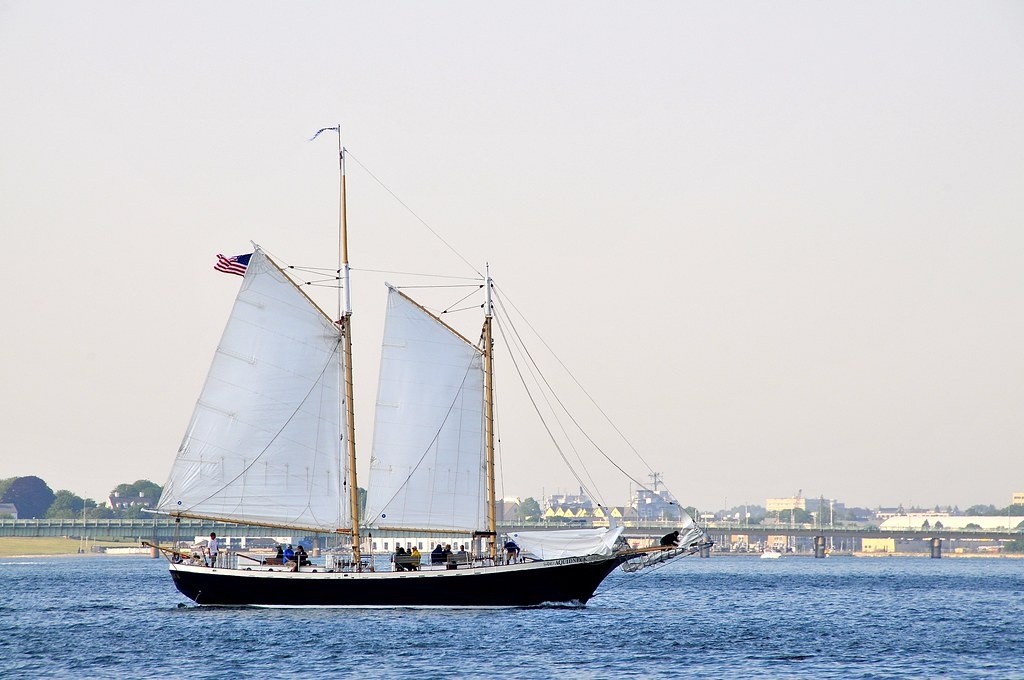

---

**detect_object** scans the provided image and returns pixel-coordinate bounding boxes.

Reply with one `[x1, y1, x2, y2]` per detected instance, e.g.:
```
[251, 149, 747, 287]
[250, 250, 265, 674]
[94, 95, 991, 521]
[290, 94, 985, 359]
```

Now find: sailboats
[141, 127, 711, 612]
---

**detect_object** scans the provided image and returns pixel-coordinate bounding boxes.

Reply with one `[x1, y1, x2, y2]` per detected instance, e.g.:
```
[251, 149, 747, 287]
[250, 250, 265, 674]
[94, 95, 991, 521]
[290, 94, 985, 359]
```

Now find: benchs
[264, 558, 298, 572]
[390, 552, 473, 572]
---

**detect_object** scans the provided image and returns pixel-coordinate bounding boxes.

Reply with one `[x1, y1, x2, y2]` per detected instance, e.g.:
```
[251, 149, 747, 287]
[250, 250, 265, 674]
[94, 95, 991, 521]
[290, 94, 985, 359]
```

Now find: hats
[276, 544, 282, 548]
[412, 546, 417, 549]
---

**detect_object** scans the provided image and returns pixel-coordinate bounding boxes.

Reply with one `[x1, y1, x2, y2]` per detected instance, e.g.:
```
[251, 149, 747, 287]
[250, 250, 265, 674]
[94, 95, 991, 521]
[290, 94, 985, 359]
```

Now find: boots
[513, 558, 518, 564]
[506, 560, 509, 565]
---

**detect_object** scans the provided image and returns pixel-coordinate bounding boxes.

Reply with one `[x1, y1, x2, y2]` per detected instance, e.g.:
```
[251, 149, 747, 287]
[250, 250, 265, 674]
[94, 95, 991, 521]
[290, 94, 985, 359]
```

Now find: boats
[761, 552, 781, 558]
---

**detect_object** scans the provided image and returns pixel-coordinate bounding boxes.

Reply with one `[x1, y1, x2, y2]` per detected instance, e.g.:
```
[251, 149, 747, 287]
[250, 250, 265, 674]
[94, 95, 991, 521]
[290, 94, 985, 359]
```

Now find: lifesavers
[173, 553, 181, 563]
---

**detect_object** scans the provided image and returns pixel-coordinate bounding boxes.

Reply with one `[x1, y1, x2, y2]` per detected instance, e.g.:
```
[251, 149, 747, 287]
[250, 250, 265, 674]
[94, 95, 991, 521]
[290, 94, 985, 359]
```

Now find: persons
[207, 532, 220, 568]
[488, 542, 496, 558]
[660, 530, 681, 551]
[390, 546, 421, 571]
[503, 541, 521, 565]
[275, 544, 313, 572]
[430, 543, 454, 563]
[458, 544, 468, 556]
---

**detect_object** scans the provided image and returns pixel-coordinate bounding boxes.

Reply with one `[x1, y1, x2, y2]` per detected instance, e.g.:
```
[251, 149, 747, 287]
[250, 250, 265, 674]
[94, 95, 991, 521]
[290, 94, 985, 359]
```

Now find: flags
[212, 253, 254, 277]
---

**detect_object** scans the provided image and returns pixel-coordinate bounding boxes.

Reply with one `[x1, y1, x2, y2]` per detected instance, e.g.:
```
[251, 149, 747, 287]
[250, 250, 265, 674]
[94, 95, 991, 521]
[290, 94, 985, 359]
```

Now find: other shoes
[668, 557, 673, 559]
[659, 560, 663, 562]
